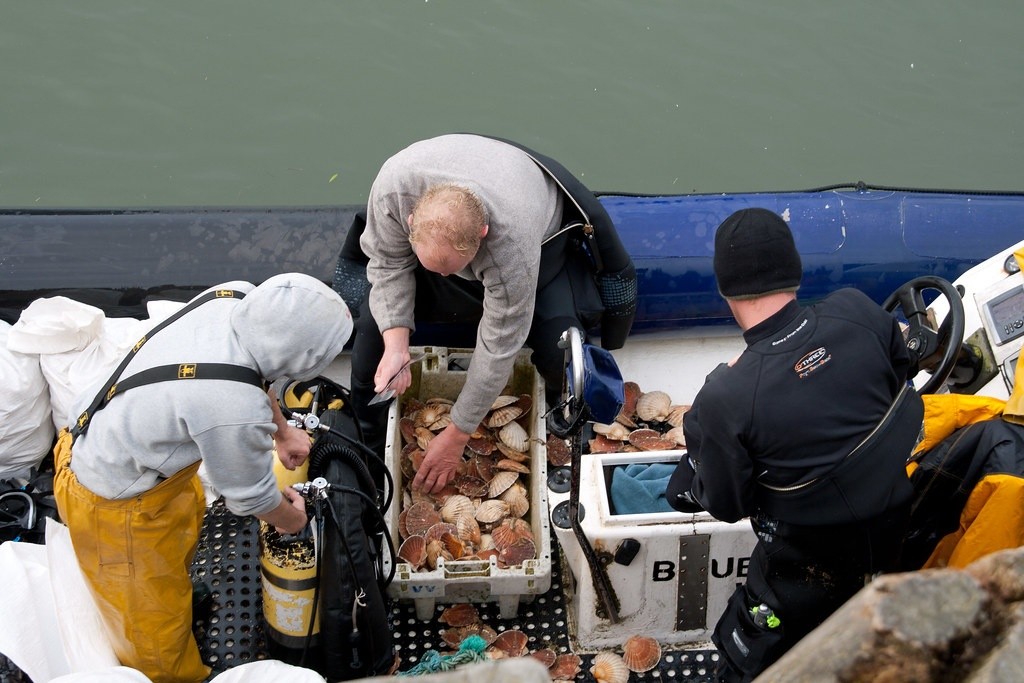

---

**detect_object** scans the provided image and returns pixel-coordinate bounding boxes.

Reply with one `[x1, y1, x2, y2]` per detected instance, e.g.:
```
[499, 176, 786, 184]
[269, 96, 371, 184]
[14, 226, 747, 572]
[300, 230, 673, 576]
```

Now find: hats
[713, 207, 803, 300]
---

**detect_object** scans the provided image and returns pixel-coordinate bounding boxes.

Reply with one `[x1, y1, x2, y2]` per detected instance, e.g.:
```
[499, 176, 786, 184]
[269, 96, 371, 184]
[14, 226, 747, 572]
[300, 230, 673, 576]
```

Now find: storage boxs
[546, 449, 758, 647]
[378, 344, 554, 618]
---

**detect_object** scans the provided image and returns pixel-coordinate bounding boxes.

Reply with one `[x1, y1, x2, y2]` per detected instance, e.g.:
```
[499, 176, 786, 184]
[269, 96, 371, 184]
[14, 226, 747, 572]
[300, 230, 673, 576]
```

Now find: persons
[50, 272, 353, 683]
[350, 132, 638, 493]
[667, 207, 924, 683]
